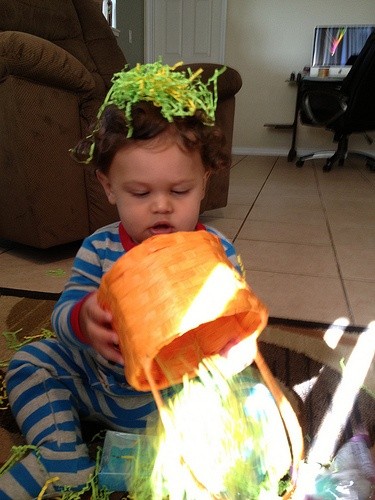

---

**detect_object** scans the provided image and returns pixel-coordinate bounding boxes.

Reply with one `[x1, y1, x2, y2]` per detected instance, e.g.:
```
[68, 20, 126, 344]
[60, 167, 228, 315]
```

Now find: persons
[0, 61, 305, 500]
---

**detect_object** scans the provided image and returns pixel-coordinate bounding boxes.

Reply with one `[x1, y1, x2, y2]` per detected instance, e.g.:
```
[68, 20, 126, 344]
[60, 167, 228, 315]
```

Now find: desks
[285, 76, 344, 164]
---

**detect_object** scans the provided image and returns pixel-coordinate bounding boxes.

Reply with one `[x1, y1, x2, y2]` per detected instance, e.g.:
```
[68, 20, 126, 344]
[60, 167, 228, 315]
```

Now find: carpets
[0, 285, 375, 499]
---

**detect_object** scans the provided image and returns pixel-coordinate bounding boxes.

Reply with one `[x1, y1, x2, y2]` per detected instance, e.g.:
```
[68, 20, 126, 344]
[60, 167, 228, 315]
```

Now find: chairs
[299, 29, 375, 173]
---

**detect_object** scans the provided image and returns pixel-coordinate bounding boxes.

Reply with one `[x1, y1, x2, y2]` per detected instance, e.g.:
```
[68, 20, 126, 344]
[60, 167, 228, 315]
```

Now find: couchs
[0, 0, 243, 249]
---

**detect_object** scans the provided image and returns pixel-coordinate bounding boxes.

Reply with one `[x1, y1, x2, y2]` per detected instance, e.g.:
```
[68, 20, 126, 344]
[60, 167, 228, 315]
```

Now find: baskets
[95, 231, 304, 499]
